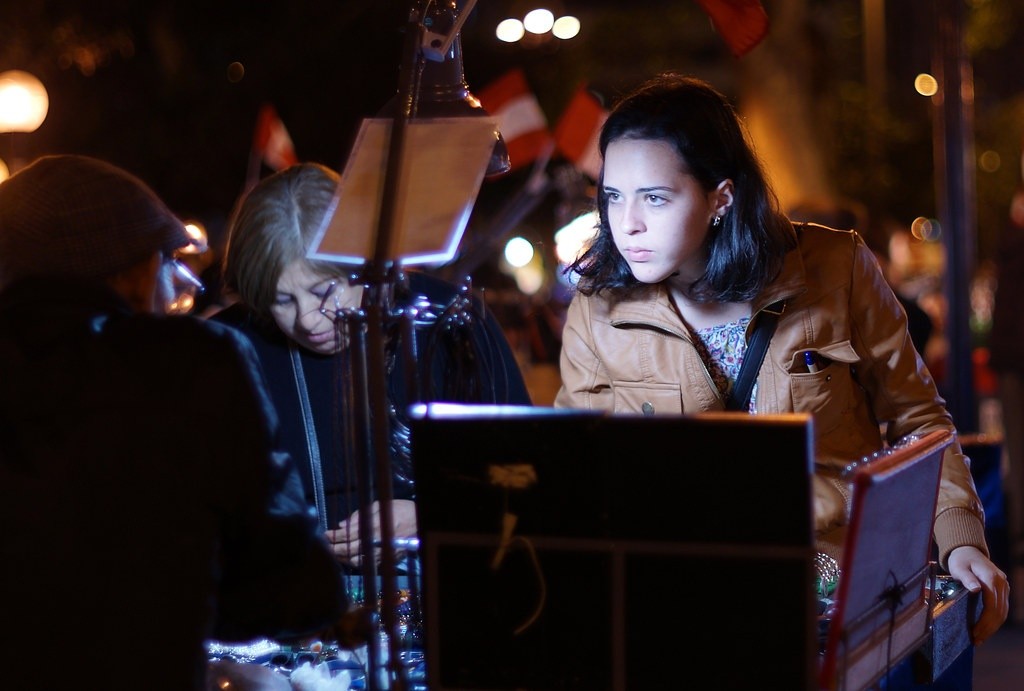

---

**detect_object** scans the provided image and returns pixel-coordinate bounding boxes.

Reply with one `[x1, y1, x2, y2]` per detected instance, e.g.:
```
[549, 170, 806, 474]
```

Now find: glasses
[165, 254, 206, 314]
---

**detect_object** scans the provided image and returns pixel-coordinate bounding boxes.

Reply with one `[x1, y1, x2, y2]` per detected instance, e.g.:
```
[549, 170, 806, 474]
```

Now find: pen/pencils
[805, 352, 818, 373]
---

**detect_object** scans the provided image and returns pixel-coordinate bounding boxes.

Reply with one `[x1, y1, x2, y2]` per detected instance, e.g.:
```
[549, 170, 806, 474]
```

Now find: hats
[0, 155, 191, 275]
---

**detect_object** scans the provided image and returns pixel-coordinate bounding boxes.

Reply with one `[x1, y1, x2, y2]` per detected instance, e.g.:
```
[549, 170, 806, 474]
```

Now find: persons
[0, 155, 347, 691]
[552, 71, 1010, 647]
[208, 162, 534, 576]
